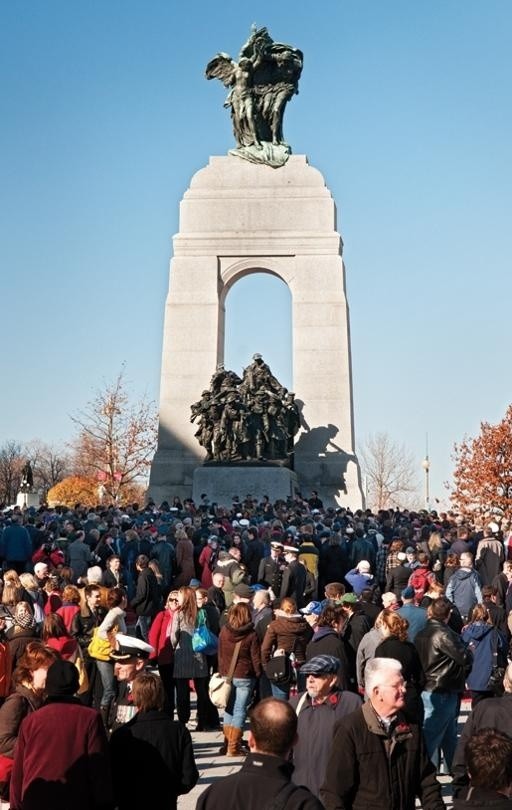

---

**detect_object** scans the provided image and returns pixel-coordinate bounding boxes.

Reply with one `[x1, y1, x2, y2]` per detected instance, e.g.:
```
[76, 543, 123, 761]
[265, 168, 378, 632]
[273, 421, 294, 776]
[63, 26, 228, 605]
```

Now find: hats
[238, 518, 250, 529]
[335, 593, 358, 606]
[270, 540, 284, 552]
[107, 632, 156, 662]
[401, 585, 417, 599]
[298, 654, 341, 676]
[282, 546, 300, 556]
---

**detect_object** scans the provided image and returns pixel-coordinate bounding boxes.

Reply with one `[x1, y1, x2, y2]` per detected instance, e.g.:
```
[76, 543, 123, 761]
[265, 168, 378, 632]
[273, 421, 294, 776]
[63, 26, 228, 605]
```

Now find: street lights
[422, 432, 432, 512]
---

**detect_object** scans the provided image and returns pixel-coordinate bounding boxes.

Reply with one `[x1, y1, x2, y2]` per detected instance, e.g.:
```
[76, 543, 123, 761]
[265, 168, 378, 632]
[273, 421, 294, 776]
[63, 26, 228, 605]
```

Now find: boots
[227, 727, 251, 757]
[219, 725, 233, 755]
[99, 704, 113, 735]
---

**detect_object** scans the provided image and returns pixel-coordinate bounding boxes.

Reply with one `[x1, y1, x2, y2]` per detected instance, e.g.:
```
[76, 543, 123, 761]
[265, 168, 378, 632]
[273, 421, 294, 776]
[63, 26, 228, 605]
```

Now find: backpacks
[411, 570, 433, 600]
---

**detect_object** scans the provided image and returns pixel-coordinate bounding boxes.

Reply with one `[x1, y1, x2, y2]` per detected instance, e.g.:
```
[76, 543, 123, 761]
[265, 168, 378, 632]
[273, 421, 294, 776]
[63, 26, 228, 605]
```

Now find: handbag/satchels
[485, 663, 507, 696]
[191, 624, 219, 655]
[208, 673, 233, 708]
[262, 656, 295, 686]
[88, 626, 118, 661]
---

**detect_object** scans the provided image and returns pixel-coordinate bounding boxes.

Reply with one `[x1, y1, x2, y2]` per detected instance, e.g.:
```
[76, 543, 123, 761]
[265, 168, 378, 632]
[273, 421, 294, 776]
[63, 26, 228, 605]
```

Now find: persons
[262, 43, 304, 144]
[191, 353, 308, 464]
[2, 487, 511, 809]
[218, 37, 266, 150]
[22, 459, 34, 488]
